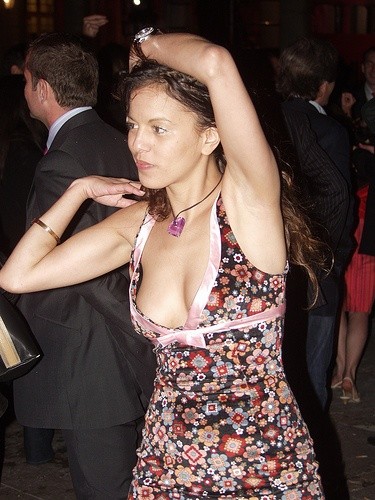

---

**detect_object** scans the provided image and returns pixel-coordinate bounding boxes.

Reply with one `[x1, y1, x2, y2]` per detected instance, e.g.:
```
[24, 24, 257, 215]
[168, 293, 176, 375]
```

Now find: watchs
[133, 25, 164, 65]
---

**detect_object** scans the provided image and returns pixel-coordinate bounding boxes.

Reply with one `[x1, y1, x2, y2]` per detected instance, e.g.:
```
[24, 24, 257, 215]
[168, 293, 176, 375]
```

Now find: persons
[1, 23, 325, 500]
[1, 13, 375, 466]
[12, 31, 156, 499]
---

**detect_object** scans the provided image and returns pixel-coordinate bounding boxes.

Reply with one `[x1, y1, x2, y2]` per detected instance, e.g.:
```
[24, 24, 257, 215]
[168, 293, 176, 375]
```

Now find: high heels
[340, 377, 361, 405]
[331, 376, 343, 392]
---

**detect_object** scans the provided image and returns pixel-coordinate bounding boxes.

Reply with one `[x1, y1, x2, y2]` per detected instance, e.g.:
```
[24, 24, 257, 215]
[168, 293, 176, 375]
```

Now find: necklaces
[162, 167, 226, 239]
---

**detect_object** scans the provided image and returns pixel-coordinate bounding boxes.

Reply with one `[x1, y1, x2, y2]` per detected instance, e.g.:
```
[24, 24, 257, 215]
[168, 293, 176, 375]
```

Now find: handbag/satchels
[0, 291, 41, 383]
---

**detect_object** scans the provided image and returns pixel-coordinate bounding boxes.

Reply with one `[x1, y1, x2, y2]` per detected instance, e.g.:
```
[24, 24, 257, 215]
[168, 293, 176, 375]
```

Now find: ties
[43, 145, 48, 157]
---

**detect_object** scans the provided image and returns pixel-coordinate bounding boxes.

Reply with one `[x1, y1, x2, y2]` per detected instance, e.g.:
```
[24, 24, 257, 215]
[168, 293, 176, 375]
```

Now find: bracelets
[31, 217, 60, 245]
